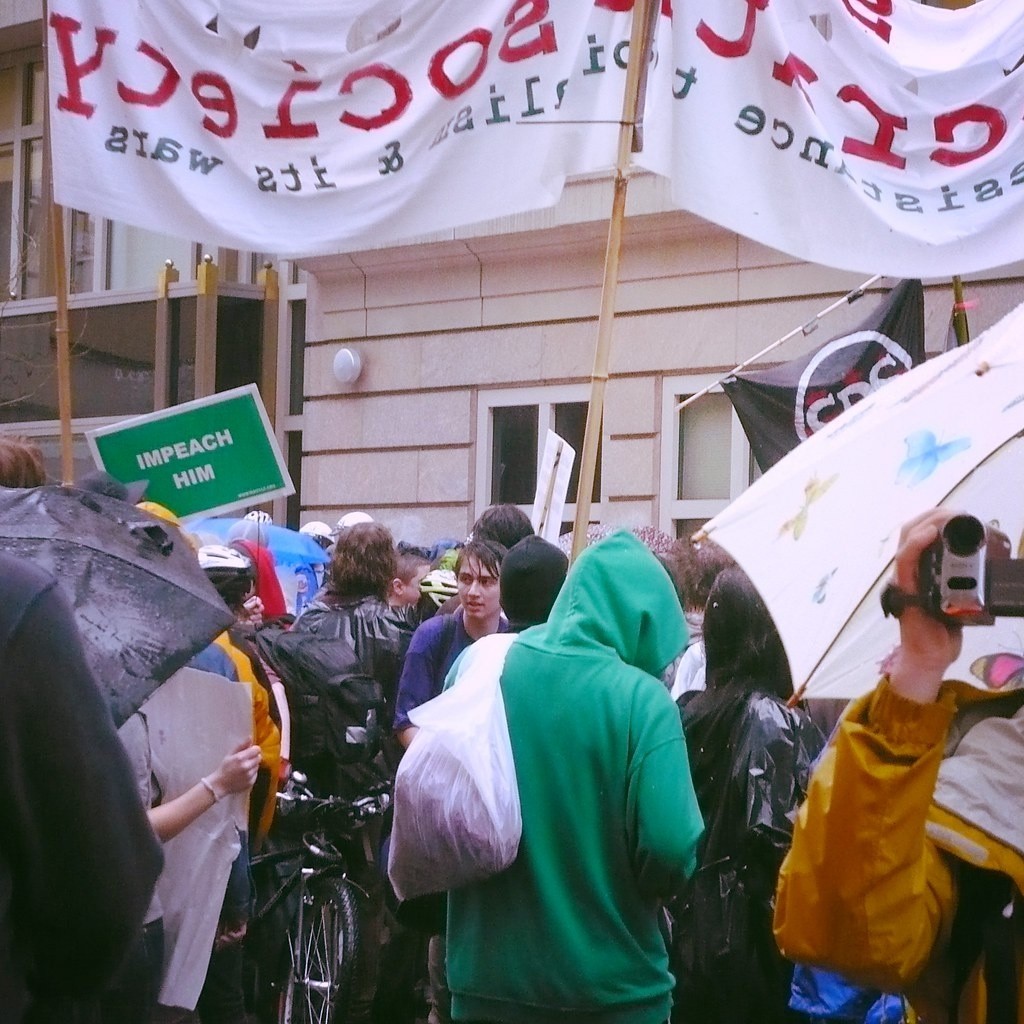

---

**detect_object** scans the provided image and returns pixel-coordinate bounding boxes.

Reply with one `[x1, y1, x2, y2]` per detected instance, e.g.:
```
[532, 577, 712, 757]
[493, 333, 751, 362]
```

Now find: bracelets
[201, 778, 220, 804]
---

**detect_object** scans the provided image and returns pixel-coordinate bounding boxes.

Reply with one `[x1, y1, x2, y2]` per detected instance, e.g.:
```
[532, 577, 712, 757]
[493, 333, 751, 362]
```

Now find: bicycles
[243, 767, 398, 1024]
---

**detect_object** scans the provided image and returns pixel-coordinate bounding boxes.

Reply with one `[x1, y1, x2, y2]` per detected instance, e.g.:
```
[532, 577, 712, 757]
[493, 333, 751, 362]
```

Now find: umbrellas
[186, 518, 331, 567]
[692, 300, 1023, 706]
[0, 485, 239, 731]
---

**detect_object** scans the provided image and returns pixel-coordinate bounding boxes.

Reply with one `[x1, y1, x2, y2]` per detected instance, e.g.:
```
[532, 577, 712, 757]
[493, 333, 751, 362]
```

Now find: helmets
[243, 509, 273, 525]
[435, 548, 460, 570]
[417, 569, 459, 608]
[134, 500, 181, 527]
[197, 544, 252, 569]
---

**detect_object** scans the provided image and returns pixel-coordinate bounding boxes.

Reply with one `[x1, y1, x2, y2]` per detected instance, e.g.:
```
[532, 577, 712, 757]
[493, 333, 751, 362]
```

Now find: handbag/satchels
[385, 633, 525, 903]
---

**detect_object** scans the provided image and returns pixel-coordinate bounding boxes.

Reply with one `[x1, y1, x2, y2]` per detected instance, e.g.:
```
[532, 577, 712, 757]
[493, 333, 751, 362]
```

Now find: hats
[329, 511, 375, 536]
[299, 521, 335, 544]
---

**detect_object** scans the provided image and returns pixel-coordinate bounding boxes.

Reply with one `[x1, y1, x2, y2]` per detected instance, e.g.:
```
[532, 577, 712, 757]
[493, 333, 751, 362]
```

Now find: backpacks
[228, 538, 290, 624]
[246, 622, 383, 768]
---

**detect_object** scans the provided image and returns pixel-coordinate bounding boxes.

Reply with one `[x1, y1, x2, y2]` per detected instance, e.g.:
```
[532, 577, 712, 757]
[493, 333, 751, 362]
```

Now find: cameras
[917, 514, 1024, 627]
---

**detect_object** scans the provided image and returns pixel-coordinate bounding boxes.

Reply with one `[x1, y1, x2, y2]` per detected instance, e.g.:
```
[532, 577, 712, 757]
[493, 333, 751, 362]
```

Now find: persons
[0, 436, 1024, 1024]
[443, 532, 705, 1024]
